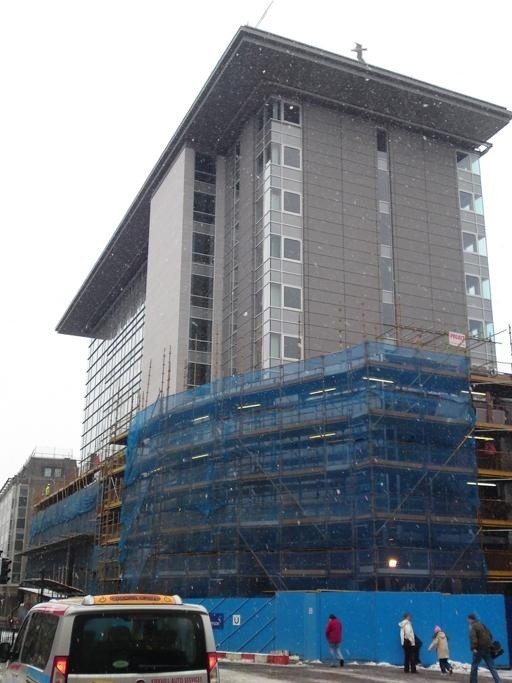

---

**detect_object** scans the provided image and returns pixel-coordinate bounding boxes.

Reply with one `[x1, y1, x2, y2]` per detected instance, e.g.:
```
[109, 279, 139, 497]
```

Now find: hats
[434, 624, 440, 632]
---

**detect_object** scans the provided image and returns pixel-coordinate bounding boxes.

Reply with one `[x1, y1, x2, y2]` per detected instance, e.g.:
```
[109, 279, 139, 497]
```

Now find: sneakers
[448, 668, 452, 676]
[411, 670, 419, 673]
[329, 665, 337, 667]
[340, 660, 344, 666]
[440, 672, 448, 676]
[403, 670, 410, 673]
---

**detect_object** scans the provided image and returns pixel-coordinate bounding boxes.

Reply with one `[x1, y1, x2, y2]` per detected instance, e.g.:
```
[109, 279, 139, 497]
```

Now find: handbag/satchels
[491, 641, 504, 658]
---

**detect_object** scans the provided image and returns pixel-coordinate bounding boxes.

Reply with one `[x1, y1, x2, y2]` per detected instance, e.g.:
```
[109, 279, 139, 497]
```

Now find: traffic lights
[0, 557, 11, 585]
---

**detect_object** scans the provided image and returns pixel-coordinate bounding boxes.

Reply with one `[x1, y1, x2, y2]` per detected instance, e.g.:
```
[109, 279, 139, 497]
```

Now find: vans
[0, 591, 219, 682]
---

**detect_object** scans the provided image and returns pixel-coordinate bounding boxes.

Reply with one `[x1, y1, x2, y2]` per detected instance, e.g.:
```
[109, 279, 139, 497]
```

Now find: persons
[467, 614, 502, 682]
[324, 614, 344, 667]
[46, 483, 52, 496]
[426, 625, 453, 677]
[397, 612, 420, 673]
